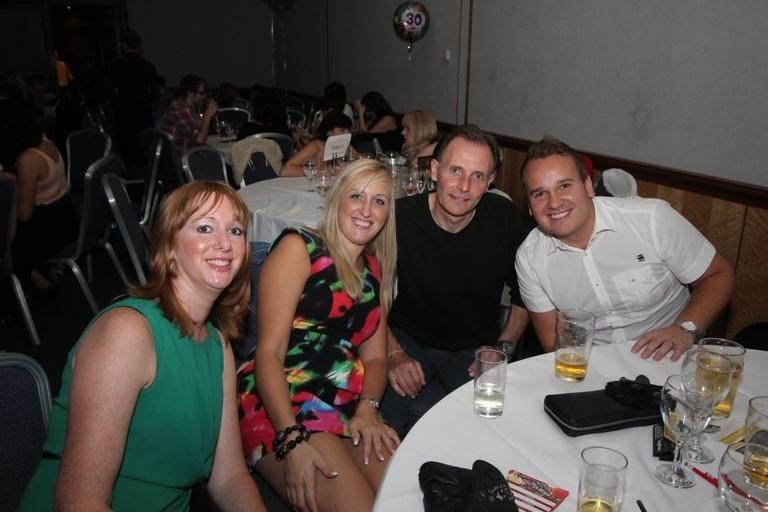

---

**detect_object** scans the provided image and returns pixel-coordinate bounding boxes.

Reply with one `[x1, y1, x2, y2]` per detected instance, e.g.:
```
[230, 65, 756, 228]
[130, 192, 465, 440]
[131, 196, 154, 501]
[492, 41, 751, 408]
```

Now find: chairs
[731, 322, 768, 349]
[592, 169, 636, 200]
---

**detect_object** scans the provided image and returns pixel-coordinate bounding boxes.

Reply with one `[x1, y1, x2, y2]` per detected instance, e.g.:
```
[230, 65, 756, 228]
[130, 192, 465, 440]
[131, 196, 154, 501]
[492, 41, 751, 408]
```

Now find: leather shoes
[28, 278, 57, 298]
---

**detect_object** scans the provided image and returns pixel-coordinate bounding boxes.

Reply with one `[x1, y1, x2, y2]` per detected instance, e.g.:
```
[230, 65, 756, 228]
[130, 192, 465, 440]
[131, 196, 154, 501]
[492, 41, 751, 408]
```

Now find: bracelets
[359, 396, 380, 408]
[387, 350, 405, 359]
[271, 424, 310, 462]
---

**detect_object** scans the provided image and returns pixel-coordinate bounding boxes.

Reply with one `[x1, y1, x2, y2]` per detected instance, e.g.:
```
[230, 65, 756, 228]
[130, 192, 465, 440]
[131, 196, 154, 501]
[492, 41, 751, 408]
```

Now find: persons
[164, 72, 439, 198]
[235, 158, 401, 512]
[383, 123, 530, 436]
[514, 134, 737, 361]
[13, 181, 267, 512]
[2, 28, 161, 305]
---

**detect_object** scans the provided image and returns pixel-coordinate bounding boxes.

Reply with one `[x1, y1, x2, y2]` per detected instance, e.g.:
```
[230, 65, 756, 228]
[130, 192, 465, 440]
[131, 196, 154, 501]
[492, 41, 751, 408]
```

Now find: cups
[472, 348, 509, 419]
[576, 446, 629, 512]
[553, 309, 597, 383]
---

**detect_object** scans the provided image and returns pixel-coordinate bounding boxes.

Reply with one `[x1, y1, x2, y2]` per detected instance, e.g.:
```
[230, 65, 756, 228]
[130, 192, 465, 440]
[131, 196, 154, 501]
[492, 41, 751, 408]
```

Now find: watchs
[493, 340, 517, 359]
[673, 319, 705, 340]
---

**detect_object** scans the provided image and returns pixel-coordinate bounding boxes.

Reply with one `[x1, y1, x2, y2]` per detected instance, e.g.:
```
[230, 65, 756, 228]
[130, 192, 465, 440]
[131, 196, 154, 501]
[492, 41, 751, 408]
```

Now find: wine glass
[652, 335, 768, 512]
[301, 148, 436, 211]
[205, 97, 251, 145]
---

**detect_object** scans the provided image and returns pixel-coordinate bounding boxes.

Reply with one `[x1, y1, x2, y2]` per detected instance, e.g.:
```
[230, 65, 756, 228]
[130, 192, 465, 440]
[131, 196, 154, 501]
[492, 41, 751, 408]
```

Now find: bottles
[372, 138, 389, 166]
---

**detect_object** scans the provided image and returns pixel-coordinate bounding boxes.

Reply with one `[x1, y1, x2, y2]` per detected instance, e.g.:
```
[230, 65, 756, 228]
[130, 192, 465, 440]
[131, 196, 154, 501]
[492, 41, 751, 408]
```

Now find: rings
[666, 340, 674, 348]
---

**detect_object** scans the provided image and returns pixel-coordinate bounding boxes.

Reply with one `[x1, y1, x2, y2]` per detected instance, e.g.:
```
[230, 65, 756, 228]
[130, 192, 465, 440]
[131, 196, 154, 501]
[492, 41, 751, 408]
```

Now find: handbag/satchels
[418, 461, 520, 512]
[541, 385, 664, 438]
